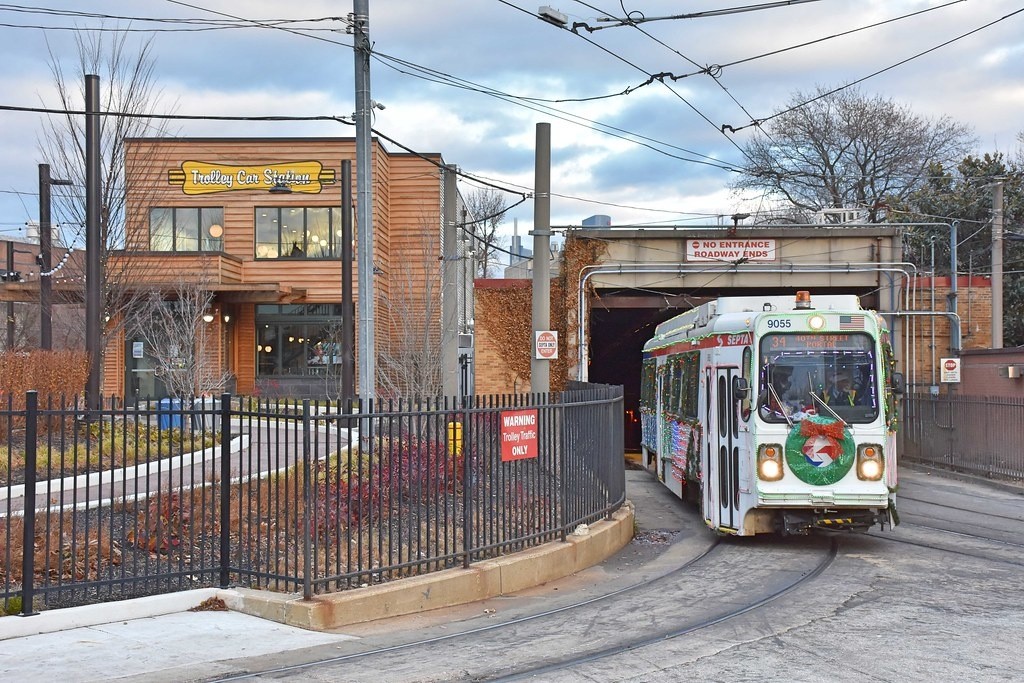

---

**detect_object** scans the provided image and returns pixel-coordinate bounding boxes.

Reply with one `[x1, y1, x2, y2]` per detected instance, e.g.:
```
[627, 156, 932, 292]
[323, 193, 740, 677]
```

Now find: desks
[308, 367, 331, 375]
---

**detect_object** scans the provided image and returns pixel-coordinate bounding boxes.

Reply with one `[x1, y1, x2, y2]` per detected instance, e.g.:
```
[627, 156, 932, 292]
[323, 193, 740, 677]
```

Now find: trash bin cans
[155, 398, 190, 433]
[192, 397, 221, 432]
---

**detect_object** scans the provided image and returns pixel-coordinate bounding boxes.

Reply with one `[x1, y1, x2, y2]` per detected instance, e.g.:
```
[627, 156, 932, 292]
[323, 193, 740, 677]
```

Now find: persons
[815, 374, 861, 406]
[315, 338, 328, 364]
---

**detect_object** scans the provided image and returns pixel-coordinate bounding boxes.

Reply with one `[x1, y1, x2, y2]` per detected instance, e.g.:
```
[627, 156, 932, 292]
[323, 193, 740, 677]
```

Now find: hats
[829, 372, 848, 383]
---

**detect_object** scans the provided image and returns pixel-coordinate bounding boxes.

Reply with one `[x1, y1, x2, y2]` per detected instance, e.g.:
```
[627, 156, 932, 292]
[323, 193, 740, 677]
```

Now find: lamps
[202, 310, 213, 322]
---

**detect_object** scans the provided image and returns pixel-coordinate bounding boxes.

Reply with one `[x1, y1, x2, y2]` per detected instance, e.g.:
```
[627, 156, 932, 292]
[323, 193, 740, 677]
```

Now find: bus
[639, 290, 904, 536]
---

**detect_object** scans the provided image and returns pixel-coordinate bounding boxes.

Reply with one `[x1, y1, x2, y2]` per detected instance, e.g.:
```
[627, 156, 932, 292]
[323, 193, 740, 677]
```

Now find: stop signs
[944, 360, 958, 371]
[537, 330, 558, 358]
[692, 240, 699, 249]
